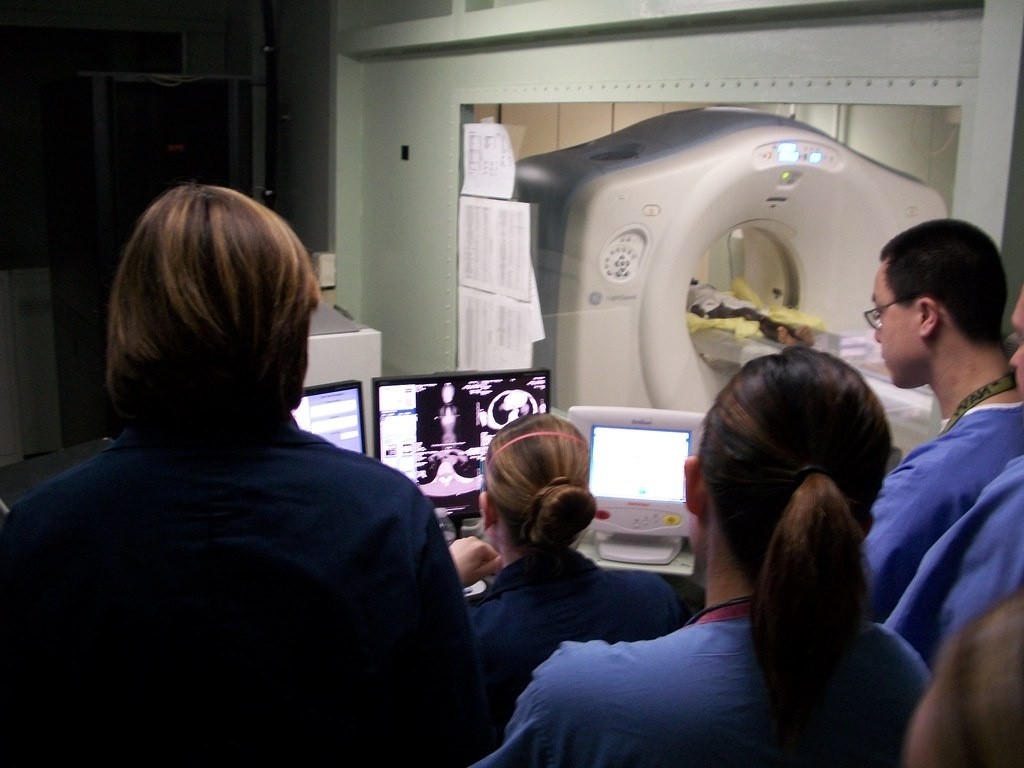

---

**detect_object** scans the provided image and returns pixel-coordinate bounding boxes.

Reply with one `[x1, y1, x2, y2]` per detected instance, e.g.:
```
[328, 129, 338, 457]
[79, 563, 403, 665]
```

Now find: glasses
[864, 295, 944, 330]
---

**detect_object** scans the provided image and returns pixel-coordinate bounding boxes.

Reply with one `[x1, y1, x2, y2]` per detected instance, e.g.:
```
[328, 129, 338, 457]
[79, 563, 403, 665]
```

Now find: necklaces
[696, 602, 752, 624]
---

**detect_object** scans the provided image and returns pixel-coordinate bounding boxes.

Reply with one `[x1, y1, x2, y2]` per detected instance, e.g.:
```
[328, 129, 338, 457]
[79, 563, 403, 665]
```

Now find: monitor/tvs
[291, 379, 367, 456]
[372, 368, 551, 541]
[567, 406, 707, 565]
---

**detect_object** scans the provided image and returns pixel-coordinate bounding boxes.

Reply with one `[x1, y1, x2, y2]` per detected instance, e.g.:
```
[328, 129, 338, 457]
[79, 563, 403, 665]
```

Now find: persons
[686, 278, 817, 347]
[865, 218, 1024, 768]
[0, 185, 497, 768]
[450, 347, 934, 768]
